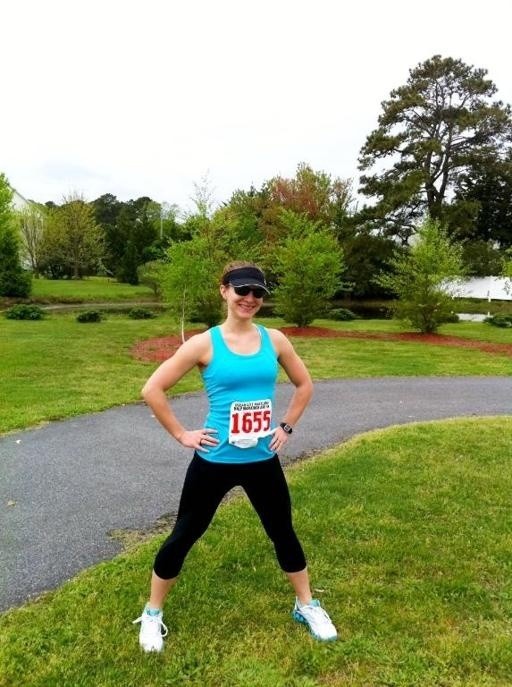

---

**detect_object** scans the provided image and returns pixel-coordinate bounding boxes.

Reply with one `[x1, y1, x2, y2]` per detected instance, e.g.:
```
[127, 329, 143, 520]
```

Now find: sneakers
[138, 601, 164, 655]
[291, 595, 339, 643]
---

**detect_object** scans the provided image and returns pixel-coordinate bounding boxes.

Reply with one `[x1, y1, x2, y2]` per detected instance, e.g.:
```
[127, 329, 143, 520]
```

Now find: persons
[131, 258, 340, 656]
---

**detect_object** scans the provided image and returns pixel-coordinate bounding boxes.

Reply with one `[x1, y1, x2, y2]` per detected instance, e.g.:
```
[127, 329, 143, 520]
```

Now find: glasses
[227, 284, 268, 299]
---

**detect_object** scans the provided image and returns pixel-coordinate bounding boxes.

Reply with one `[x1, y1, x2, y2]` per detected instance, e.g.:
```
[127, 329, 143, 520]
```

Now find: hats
[221, 267, 271, 296]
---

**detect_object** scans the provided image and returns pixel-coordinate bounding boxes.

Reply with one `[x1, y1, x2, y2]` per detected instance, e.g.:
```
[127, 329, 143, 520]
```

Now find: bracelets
[279, 422, 293, 434]
